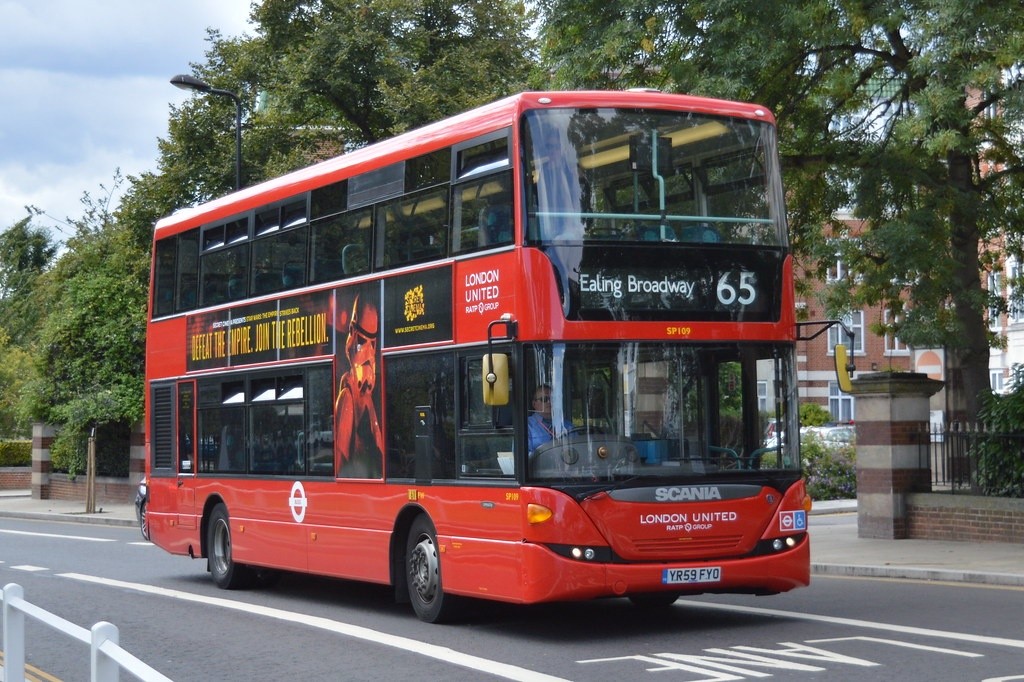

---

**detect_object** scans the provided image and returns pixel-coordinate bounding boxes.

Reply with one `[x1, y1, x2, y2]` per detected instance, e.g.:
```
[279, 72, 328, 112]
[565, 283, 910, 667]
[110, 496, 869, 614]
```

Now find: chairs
[169, 189, 719, 307]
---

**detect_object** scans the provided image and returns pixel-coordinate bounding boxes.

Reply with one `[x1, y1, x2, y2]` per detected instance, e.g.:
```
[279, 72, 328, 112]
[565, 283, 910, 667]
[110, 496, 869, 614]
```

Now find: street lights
[169, 72, 243, 193]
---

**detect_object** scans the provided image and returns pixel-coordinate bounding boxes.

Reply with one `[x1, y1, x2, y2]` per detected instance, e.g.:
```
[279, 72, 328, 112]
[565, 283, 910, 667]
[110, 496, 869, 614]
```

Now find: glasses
[536, 395, 550, 403]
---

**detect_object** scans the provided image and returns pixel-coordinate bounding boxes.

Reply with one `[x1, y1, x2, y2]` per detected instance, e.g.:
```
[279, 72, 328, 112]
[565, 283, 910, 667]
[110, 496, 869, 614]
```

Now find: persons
[528, 384, 578, 451]
[432, 371, 492, 479]
[335, 293, 381, 476]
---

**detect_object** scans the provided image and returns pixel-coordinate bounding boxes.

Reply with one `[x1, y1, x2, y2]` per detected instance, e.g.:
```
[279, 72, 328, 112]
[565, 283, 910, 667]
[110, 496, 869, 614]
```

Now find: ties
[548, 422, 554, 439]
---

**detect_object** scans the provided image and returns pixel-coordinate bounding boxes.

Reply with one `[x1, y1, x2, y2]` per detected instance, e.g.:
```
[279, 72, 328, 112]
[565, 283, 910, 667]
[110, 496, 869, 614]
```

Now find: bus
[145, 86, 857, 616]
